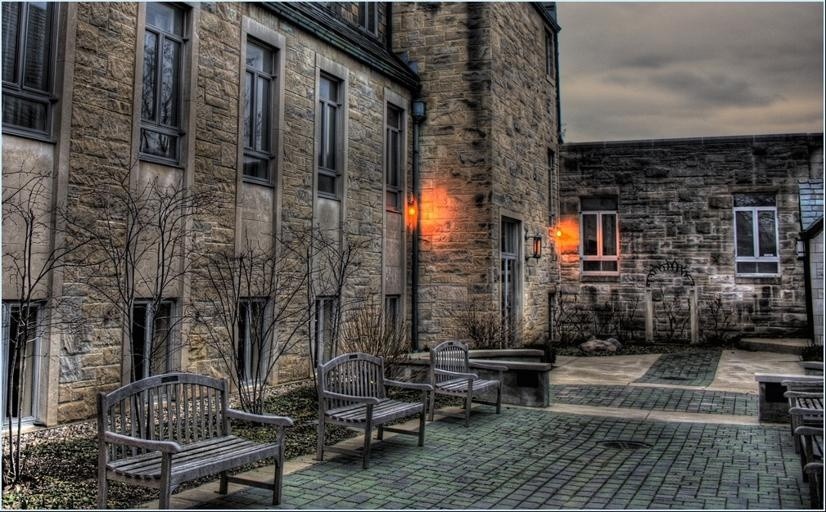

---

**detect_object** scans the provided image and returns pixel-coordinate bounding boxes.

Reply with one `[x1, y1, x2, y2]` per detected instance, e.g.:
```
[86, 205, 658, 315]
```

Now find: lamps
[524, 232, 542, 263]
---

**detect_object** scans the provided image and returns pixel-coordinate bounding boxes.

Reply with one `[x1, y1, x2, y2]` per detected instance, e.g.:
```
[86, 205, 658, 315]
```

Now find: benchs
[427, 341, 504, 430]
[95, 372, 295, 508]
[313, 349, 436, 470]
